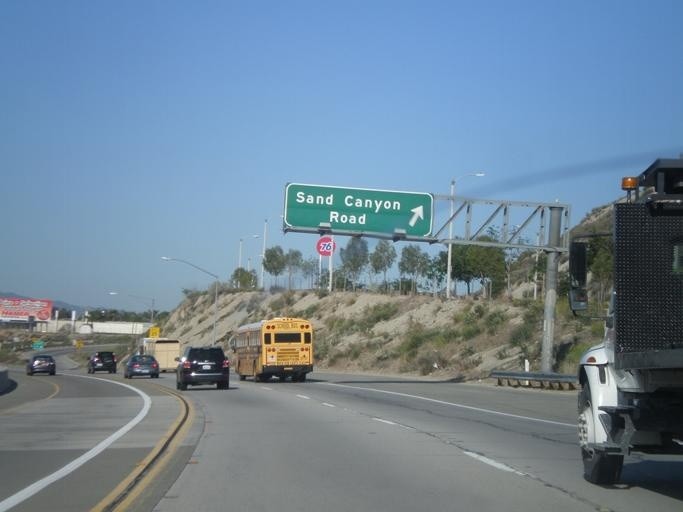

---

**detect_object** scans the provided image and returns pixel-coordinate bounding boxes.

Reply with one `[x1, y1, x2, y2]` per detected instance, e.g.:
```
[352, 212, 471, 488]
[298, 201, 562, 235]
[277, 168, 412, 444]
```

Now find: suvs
[86, 351, 116, 374]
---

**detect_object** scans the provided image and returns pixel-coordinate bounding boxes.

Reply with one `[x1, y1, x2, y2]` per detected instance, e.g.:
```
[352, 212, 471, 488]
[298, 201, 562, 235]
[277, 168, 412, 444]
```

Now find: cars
[25, 354, 55, 375]
[124, 355, 159, 379]
[173, 345, 229, 390]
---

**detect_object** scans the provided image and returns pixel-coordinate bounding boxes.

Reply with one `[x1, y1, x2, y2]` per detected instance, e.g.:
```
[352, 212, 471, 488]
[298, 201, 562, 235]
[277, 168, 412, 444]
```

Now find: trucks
[567, 179, 682, 485]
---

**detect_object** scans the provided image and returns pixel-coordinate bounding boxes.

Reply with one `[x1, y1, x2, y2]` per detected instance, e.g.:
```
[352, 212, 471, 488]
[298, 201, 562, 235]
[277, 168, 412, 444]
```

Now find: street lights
[445, 172, 485, 298]
[236, 215, 275, 289]
[107, 291, 155, 323]
[160, 256, 218, 346]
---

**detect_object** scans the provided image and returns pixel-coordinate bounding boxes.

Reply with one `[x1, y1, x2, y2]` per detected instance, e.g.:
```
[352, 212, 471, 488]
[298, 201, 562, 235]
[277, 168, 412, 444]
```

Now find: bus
[227, 317, 313, 382]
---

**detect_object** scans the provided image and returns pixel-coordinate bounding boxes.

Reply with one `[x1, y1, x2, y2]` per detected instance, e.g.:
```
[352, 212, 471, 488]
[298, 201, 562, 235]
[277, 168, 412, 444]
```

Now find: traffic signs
[281, 183, 433, 241]
